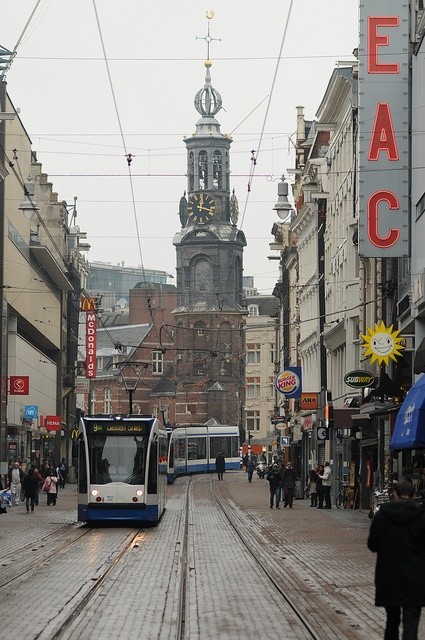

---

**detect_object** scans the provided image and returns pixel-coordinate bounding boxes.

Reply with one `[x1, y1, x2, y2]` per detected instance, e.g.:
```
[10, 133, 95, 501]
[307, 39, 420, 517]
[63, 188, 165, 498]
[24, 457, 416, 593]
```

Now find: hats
[394, 480, 416, 499]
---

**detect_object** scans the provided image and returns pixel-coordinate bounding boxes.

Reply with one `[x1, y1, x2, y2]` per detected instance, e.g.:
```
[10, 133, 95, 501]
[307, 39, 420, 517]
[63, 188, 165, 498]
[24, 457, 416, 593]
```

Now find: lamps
[17, 173, 40, 221]
[270, 173, 297, 222]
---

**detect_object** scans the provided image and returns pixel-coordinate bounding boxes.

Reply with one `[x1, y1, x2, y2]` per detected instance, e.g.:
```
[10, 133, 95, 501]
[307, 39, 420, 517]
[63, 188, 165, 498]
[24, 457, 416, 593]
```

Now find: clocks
[230, 193, 239, 225]
[185, 192, 216, 226]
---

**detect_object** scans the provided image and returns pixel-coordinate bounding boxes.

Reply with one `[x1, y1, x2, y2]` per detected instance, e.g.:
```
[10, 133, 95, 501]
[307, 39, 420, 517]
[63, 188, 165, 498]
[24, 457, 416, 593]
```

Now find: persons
[317, 465, 324, 509]
[367, 480, 425, 640]
[246, 458, 254, 482]
[307, 470, 318, 507]
[23, 457, 69, 513]
[280, 462, 297, 509]
[216, 452, 225, 480]
[266, 464, 282, 509]
[8, 461, 23, 506]
[318, 462, 332, 509]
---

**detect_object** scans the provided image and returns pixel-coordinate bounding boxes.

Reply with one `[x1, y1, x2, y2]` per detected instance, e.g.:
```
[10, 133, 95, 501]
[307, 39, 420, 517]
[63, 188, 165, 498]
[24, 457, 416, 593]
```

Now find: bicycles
[335, 475, 349, 510]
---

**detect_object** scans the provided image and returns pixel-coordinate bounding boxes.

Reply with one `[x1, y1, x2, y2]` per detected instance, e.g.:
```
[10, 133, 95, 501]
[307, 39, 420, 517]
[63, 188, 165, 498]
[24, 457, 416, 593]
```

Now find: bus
[74, 413, 243, 528]
